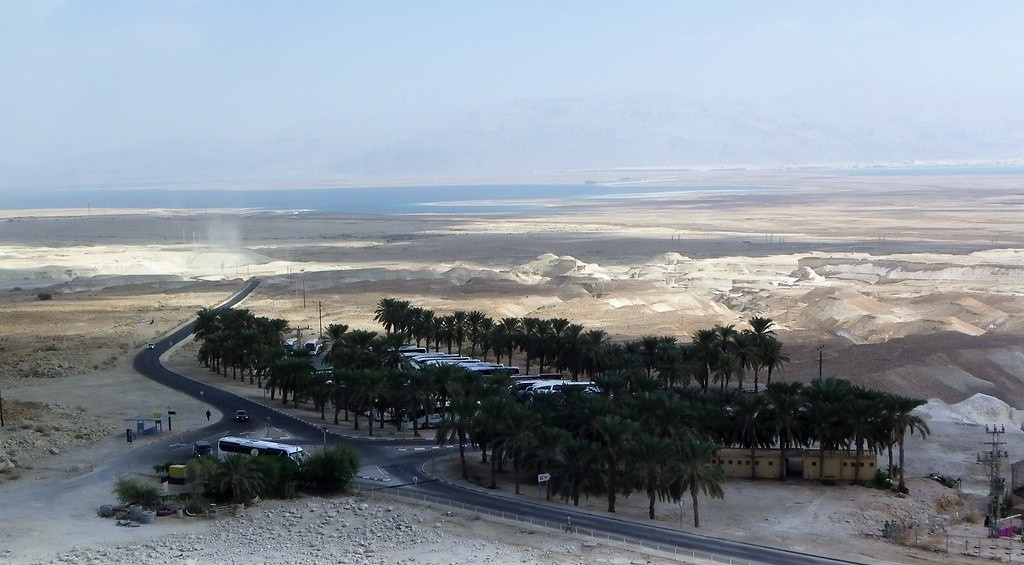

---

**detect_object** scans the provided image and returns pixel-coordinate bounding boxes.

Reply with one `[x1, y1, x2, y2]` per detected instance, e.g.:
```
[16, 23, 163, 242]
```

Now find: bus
[302, 339, 320, 356]
[368, 346, 598, 395]
[217, 436, 310, 471]
[283, 337, 297, 355]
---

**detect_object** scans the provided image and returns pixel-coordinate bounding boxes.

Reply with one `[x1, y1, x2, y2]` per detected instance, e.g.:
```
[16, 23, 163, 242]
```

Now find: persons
[206, 409, 211, 421]
[565, 512, 572, 534]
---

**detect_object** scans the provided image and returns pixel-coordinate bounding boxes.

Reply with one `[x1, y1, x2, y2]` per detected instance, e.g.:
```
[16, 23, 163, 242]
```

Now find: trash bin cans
[176, 508, 183, 519]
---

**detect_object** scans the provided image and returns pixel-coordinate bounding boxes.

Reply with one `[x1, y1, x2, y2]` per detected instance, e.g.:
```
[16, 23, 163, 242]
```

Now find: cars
[147, 343, 154, 348]
[234, 409, 249, 422]
[193, 440, 213, 459]
